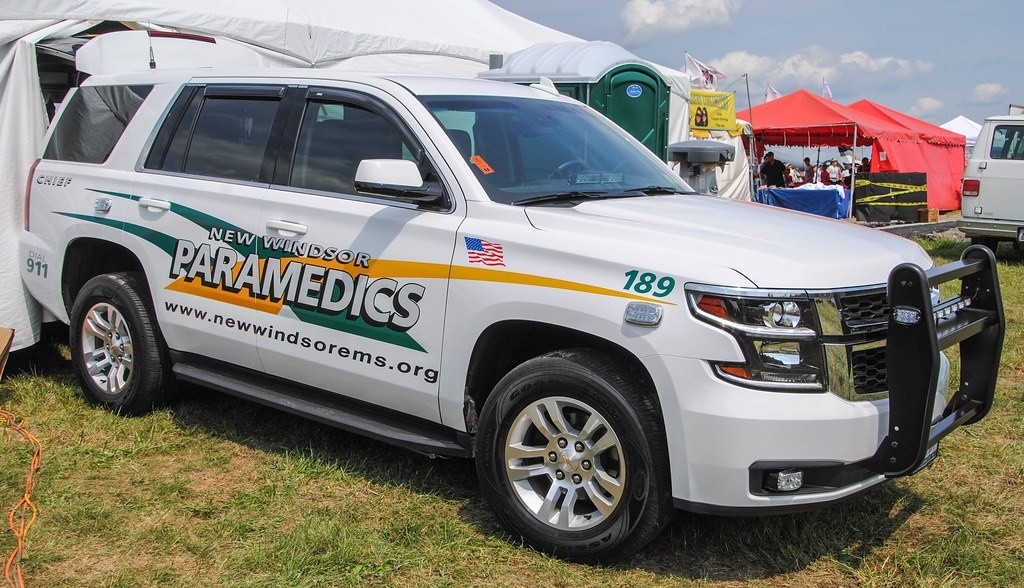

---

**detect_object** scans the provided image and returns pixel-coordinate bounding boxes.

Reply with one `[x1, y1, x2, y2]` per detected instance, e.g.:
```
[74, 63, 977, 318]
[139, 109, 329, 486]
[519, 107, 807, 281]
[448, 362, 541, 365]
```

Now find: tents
[1, 0, 757, 353]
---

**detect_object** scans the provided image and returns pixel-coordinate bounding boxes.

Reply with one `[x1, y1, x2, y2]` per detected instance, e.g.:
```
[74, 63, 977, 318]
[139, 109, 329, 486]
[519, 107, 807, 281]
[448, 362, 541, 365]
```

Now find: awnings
[938, 116, 1007, 149]
[736, 92, 965, 215]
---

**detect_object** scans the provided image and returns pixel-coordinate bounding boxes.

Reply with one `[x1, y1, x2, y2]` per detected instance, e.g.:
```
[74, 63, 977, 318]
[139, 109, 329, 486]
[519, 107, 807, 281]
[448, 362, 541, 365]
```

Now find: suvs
[21, 58, 1008, 565]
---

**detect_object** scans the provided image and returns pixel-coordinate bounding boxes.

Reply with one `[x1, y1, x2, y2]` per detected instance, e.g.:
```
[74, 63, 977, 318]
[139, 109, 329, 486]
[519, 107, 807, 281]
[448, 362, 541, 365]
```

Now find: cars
[955, 109, 1024, 263]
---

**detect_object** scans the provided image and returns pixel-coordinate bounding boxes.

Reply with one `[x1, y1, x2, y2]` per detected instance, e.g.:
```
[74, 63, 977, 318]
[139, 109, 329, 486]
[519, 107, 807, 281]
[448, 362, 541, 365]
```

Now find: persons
[757, 152, 791, 187]
[803, 157, 814, 182]
[813, 163, 824, 183]
[820, 165, 830, 183]
[760, 154, 771, 184]
[858, 157, 869, 171]
[828, 159, 842, 182]
[782, 160, 803, 186]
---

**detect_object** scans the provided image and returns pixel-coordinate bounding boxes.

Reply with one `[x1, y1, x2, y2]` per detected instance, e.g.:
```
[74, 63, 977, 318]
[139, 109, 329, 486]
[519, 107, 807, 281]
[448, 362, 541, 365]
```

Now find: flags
[767, 85, 781, 102]
[685, 53, 728, 92]
[823, 81, 832, 101]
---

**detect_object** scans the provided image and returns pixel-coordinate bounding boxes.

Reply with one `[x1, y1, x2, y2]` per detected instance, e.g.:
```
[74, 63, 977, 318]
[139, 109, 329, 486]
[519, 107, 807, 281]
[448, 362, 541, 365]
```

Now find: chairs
[186, 114, 247, 178]
[304, 119, 375, 200]
[416, 129, 472, 195]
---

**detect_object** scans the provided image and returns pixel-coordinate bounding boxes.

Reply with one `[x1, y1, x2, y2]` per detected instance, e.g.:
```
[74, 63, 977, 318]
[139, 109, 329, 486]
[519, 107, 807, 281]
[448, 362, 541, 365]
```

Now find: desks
[757, 186, 850, 222]
